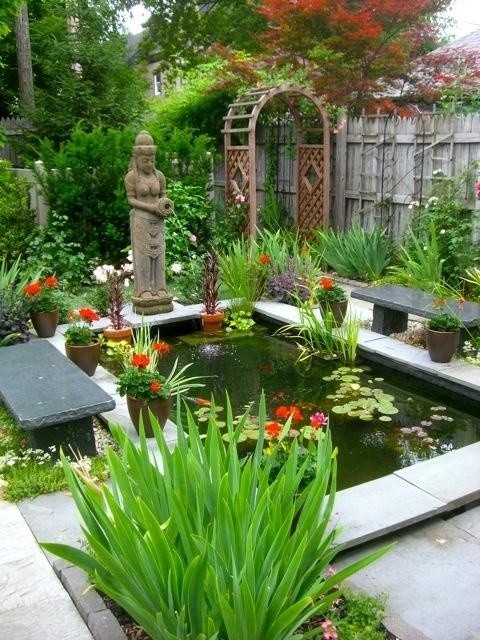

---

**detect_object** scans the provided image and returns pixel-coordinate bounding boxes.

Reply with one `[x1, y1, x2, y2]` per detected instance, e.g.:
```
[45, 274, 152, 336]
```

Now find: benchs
[0, 338, 117, 461]
[352, 283, 479, 355]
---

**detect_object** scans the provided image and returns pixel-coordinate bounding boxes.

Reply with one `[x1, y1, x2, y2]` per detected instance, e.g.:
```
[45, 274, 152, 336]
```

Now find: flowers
[62, 308, 99, 343]
[118, 346, 179, 398]
[316, 277, 347, 298]
[20, 274, 62, 310]
[258, 253, 269, 266]
[255, 406, 339, 489]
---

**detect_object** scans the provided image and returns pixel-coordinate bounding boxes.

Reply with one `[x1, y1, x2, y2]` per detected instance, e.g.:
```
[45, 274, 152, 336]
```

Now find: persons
[124, 129, 177, 306]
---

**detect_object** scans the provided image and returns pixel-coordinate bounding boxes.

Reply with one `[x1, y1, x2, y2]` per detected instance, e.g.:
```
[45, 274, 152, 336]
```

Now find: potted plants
[424, 314, 460, 363]
[102, 270, 133, 348]
[200, 252, 224, 332]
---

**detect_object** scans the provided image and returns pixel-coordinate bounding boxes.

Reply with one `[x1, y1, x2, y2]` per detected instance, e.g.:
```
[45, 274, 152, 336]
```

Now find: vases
[318, 298, 350, 327]
[276, 493, 308, 535]
[126, 399, 174, 439]
[65, 343, 100, 376]
[29, 311, 59, 336]
[242, 272, 266, 301]
